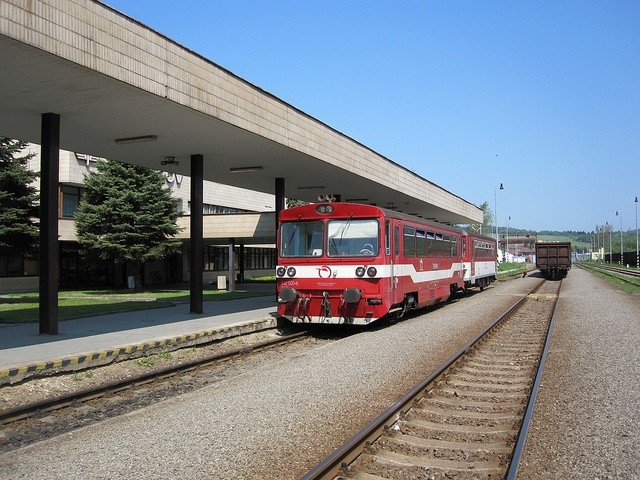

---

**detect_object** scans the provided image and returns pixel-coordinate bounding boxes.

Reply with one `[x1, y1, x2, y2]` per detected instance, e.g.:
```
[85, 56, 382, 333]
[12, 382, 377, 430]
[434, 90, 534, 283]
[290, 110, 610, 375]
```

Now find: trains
[535, 241, 571, 278]
[276, 195, 495, 332]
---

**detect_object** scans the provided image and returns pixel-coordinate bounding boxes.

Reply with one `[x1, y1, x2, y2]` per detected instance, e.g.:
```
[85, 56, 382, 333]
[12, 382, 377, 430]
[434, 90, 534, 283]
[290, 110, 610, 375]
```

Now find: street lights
[616, 211, 623, 266]
[496, 183, 504, 263]
[606, 221, 612, 264]
[506, 216, 511, 262]
[634, 196, 640, 268]
